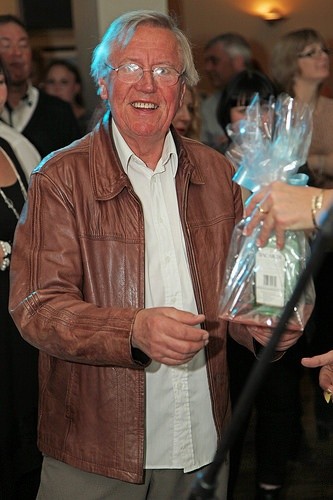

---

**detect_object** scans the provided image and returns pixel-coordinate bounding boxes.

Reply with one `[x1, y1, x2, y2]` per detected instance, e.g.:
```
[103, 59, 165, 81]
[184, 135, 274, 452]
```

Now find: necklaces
[0, 146, 28, 220]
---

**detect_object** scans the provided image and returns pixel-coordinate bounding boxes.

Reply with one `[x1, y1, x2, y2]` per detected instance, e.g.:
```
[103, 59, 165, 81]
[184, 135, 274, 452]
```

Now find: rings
[257, 204, 269, 214]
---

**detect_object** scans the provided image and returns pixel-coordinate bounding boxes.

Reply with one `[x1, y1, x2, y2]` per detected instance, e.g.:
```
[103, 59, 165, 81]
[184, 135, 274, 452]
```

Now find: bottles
[252, 233, 301, 318]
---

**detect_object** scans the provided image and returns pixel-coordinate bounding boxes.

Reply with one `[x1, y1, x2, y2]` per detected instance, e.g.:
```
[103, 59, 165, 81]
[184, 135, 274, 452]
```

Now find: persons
[266, 27, 333, 440]
[0, 15, 81, 160]
[45, 59, 94, 135]
[199, 31, 258, 155]
[171, 84, 202, 143]
[301, 350, 333, 404]
[8, 10, 303, 500]
[215, 69, 319, 500]
[0, 58, 42, 500]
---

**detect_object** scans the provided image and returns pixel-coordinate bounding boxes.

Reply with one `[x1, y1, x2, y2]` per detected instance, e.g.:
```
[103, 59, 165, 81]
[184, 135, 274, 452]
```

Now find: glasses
[105, 62, 184, 87]
[298, 46, 330, 57]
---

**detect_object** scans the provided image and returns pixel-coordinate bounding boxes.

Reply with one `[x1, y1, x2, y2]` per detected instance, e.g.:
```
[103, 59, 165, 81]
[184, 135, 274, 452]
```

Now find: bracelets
[0, 241, 7, 271]
[320, 155, 325, 175]
[311, 189, 324, 230]
